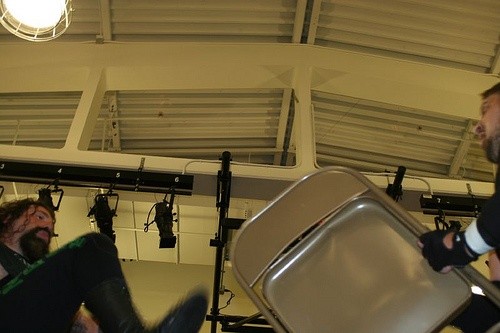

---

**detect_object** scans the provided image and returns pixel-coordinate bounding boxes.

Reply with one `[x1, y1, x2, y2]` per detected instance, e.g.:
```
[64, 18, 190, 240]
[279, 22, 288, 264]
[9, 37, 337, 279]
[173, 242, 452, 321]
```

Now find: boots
[84, 278, 207, 333]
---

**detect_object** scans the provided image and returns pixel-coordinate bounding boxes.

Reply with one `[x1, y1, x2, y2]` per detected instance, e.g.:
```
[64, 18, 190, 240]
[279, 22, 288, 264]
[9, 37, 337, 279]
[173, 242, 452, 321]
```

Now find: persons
[0, 198, 209, 333]
[419, 80, 500, 333]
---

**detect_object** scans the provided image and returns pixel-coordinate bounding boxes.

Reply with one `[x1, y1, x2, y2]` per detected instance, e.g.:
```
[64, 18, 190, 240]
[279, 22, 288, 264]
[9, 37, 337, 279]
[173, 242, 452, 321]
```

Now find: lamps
[0, 1, 73, 42]
[144, 190, 178, 249]
[36, 184, 63, 236]
[90, 183, 121, 244]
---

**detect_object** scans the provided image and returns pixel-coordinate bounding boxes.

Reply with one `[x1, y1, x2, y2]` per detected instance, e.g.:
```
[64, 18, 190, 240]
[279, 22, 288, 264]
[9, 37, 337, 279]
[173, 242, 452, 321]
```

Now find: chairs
[231, 166, 500, 333]
